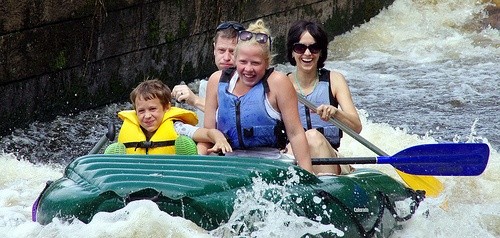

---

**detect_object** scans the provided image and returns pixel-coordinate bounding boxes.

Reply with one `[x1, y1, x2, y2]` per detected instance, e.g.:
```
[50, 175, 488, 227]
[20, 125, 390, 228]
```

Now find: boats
[30, 150, 427, 238]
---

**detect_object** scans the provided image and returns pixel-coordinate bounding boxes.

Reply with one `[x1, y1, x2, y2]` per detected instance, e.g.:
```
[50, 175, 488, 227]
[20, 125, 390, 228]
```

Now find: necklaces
[294, 69, 318, 97]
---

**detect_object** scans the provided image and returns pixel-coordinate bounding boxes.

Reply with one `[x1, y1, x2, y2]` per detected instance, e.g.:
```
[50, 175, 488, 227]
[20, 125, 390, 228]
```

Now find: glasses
[214, 23, 245, 33]
[292, 43, 321, 54]
[237, 30, 272, 52]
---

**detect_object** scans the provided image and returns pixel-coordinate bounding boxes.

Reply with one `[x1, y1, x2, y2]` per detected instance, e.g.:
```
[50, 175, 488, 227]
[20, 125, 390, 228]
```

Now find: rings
[181, 92, 183, 95]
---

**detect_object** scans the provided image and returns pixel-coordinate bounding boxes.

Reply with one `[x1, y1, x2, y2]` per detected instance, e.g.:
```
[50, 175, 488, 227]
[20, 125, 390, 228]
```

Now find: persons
[282, 21, 362, 176]
[104, 79, 232, 155]
[171, 21, 246, 113]
[197, 20, 313, 174]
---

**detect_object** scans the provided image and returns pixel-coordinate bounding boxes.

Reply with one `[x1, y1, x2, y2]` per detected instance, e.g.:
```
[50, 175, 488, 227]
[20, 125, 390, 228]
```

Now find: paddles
[296, 92, 445, 198]
[32, 124, 116, 222]
[311, 143, 490, 176]
